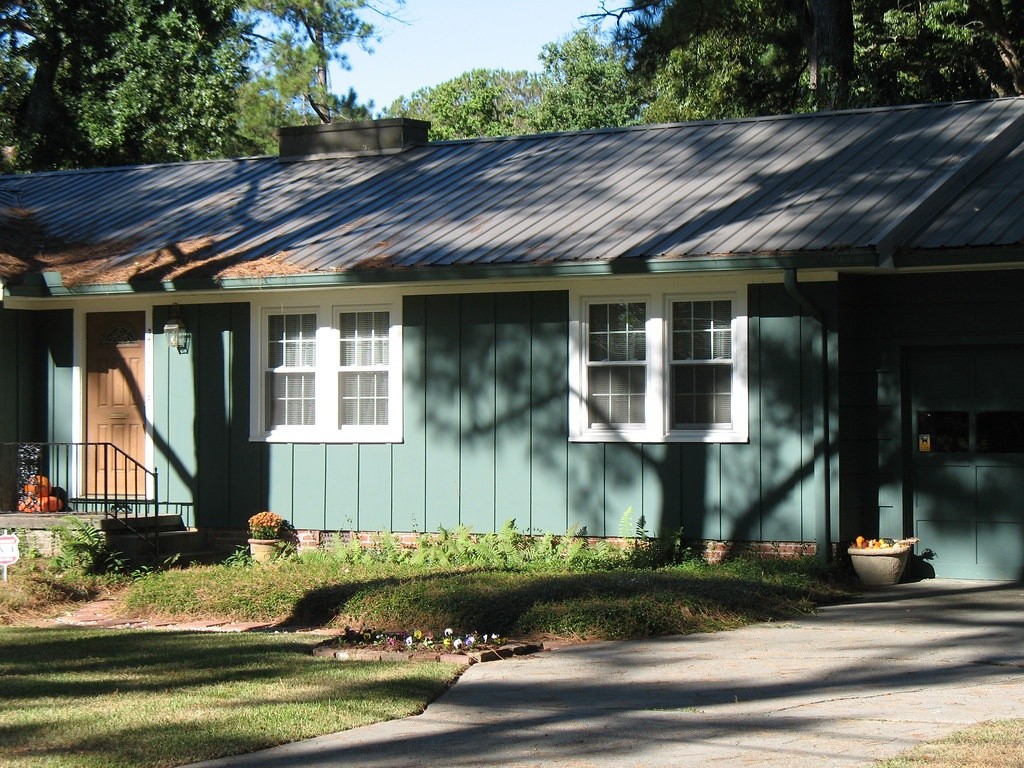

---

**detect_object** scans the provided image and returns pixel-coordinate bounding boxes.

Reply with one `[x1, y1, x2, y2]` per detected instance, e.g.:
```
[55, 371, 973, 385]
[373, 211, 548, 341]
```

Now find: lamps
[163, 319, 186, 348]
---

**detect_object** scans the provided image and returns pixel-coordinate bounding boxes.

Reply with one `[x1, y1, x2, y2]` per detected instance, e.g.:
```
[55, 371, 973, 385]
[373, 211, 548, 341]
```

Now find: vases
[848, 545, 911, 586]
[248, 538, 281, 564]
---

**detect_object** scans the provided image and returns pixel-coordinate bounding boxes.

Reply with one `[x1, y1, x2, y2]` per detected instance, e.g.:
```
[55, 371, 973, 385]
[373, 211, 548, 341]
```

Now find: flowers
[247, 511, 282, 540]
